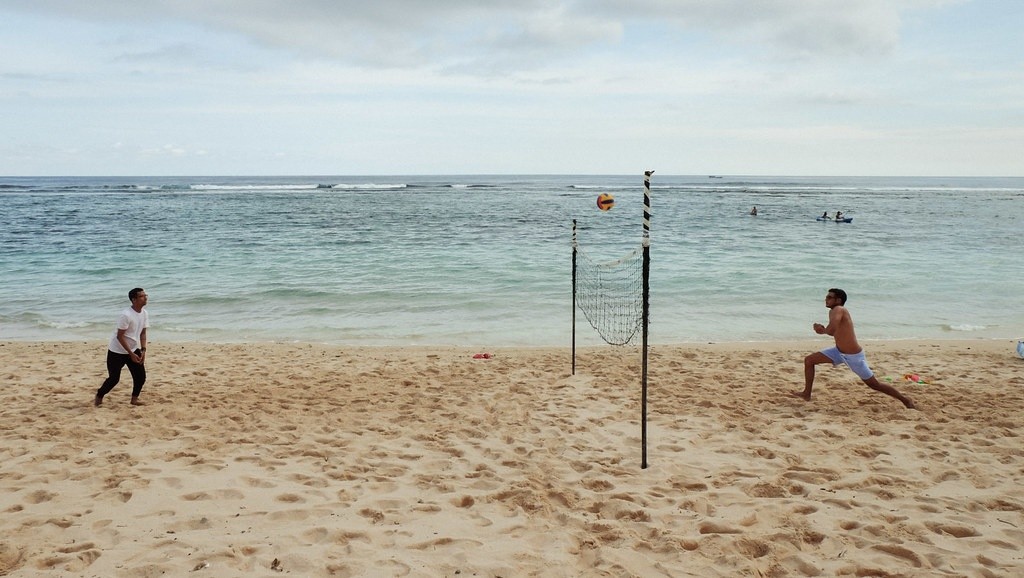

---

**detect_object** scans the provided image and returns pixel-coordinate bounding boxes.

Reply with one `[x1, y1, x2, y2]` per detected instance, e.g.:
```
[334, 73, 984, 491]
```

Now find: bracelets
[141, 347, 146, 352]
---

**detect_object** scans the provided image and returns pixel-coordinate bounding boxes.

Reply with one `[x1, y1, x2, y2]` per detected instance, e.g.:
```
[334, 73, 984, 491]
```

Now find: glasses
[138, 294, 148, 298]
[826, 295, 838, 300]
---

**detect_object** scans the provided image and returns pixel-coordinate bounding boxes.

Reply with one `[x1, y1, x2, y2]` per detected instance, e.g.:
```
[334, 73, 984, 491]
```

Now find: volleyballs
[596, 193, 615, 211]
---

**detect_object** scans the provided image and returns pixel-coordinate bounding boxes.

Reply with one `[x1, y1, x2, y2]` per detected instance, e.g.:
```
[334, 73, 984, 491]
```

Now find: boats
[816, 215, 853, 223]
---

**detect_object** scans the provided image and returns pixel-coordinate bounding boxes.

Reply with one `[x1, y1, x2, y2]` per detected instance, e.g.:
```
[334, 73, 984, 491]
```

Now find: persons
[821, 211, 831, 219]
[95, 288, 150, 408]
[790, 288, 916, 409]
[750, 206, 757, 215]
[836, 211, 844, 219]
